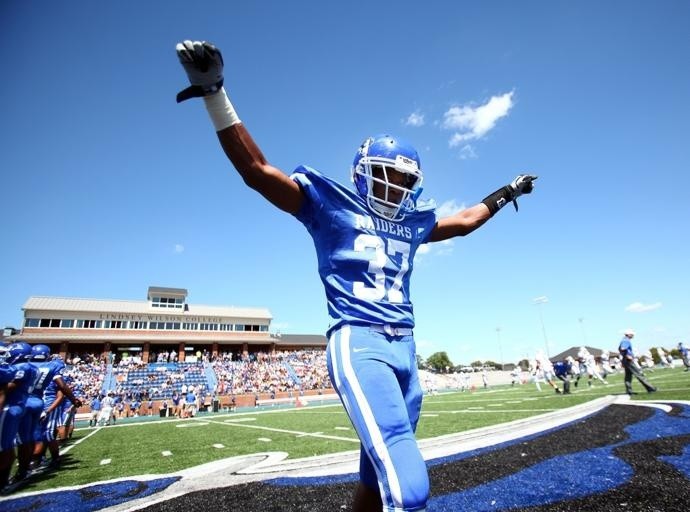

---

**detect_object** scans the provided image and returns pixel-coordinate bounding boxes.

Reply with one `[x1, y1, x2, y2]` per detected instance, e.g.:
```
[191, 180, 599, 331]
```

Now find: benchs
[115, 360, 219, 404]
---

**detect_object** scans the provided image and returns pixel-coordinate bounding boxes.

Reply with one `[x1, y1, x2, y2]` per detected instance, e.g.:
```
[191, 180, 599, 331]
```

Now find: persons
[84, 346, 337, 428]
[174, 37, 541, 511]
[425, 328, 689, 394]
[0, 340, 84, 494]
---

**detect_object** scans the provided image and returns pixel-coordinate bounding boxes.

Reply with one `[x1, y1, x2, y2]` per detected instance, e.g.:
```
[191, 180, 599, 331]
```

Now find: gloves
[504, 174, 538, 200]
[175, 40, 224, 104]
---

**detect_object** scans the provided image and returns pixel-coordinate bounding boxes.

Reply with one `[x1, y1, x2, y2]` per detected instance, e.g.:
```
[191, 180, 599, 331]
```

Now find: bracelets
[205, 85, 240, 133]
[483, 186, 512, 218]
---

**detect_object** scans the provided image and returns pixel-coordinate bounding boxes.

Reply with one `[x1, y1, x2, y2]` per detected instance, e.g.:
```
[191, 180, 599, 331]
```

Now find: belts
[369, 324, 413, 338]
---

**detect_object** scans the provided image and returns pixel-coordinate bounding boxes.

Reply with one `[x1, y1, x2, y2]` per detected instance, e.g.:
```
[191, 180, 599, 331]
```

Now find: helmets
[64, 377, 71, 383]
[0, 342, 7, 355]
[5, 342, 33, 365]
[352, 133, 423, 223]
[29, 345, 50, 362]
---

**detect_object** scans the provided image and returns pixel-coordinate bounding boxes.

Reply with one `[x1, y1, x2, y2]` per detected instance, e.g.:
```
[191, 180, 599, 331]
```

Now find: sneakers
[536, 379, 609, 395]
[89, 422, 116, 429]
[0, 437, 73, 496]
[648, 387, 657, 393]
[629, 392, 638, 396]
[177, 415, 194, 420]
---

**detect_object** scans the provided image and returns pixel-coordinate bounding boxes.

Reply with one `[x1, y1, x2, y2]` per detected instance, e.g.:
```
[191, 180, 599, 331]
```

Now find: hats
[624, 329, 635, 335]
